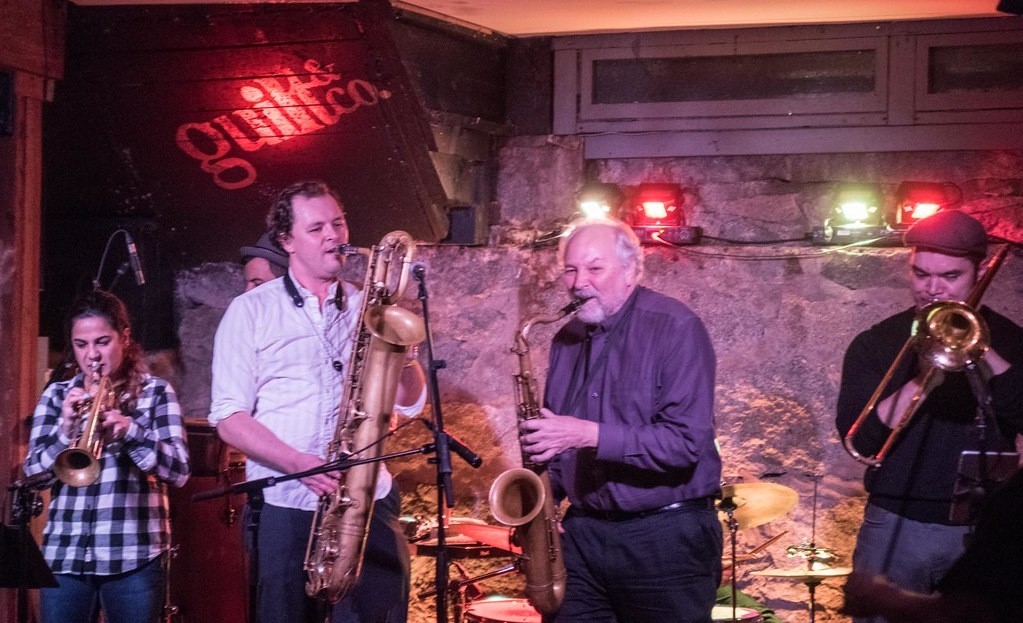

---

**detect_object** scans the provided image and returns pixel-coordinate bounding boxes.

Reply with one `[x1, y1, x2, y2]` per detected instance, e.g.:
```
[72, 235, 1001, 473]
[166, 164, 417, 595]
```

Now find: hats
[239, 221, 288, 268]
[903, 210, 988, 258]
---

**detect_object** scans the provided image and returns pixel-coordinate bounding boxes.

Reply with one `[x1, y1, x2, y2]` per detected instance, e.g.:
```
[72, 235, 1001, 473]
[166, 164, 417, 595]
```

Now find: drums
[178, 416, 228, 476]
[215, 462, 246, 527]
[465, 596, 541, 623]
[710, 604, 763, 623]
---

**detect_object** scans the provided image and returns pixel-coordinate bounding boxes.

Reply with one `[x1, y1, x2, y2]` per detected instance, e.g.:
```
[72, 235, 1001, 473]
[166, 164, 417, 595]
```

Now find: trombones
[845, 239, 1014, 469]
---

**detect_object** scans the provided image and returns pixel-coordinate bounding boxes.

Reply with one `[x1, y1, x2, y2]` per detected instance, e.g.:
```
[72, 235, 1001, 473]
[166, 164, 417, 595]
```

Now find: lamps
[634, 181, 684, 224]
[575, 184, 627, 218]
[892, 178, 950, 227]
[827, 177, 884, 227]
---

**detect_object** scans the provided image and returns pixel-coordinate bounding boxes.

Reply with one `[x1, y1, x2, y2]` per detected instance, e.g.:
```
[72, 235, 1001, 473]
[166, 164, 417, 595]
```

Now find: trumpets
[54, 360, 114, 487]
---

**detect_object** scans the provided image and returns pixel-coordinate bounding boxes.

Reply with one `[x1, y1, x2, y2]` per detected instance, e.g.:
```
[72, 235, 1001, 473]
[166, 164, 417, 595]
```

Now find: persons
[209, 175, 429, 623]
[518, 217, 726, 623]
[831, 207, 1023, 622]
[237, 232, 314, 623]
[20, 287, 187, 623]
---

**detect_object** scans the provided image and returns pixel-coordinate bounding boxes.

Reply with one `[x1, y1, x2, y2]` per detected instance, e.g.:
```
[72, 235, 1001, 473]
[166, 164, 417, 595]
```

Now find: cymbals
[747, 566, 853, 578]
[713, 481, 798, 533]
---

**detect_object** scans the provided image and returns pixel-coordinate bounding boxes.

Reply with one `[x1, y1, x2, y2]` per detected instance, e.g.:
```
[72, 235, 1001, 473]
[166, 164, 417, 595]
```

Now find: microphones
[962, 351, 993, 408]
[411, 261, 429, 301]
[125, 232, 145, 286]
[428, 421, 482, 469]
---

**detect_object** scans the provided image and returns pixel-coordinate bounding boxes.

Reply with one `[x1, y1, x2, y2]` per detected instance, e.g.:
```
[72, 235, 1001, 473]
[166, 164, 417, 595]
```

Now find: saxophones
[489, 296, 586, 615]
[302, 227, 427, 608]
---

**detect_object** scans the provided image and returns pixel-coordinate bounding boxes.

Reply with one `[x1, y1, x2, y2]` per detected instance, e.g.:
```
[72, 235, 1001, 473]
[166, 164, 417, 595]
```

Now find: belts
[569, 496, 714, 522]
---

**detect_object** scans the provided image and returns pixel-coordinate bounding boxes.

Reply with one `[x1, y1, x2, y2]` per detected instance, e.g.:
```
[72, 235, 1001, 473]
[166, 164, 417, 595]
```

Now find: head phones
[283, 265, 343, 310]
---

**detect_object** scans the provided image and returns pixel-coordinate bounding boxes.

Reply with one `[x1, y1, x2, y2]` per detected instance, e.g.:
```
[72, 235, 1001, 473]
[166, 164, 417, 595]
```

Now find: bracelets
[401, 345, 420, 370]
